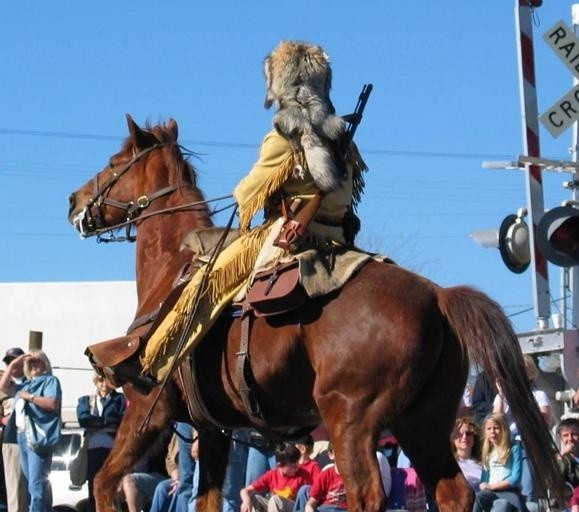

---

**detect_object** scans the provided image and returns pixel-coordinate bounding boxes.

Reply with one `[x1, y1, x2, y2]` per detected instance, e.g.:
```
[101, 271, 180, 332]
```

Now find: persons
[450, 354, 578, 511]
[0, 349, 62, 512]
[376, 448, 438, 510]
[78, 372, 125, 511]
[124, 422, 348, 511]
[85, 41, 369, 395]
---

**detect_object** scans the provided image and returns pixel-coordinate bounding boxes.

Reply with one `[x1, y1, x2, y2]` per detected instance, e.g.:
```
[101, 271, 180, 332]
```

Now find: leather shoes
[87, 340, 161, 397]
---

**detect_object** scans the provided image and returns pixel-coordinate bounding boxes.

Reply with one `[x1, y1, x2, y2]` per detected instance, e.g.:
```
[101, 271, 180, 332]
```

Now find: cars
[45, 424, 90, 505]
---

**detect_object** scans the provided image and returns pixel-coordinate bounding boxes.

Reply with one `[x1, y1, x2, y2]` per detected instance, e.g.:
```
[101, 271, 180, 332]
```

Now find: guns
[272, 84, 375, 251]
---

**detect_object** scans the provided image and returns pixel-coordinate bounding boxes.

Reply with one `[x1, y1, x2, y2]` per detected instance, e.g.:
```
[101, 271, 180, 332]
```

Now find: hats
[3, 347, 24, 361]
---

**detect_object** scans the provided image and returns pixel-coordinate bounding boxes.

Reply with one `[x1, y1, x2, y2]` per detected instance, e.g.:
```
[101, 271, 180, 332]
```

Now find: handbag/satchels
[26, 416, 62, 452]
[68, 447, 89, 486]
[245, 260, 309, 318]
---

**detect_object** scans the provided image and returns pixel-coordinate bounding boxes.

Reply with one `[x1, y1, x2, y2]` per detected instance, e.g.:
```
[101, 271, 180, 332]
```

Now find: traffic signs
[537, 17, 579, 138]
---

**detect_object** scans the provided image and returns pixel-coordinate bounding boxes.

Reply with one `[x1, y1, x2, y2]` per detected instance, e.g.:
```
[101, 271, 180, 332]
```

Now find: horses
[67, 113, 569, 512]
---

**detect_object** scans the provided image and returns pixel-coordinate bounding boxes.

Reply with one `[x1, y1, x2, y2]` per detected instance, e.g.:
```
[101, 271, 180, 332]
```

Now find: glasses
[455, 431, 475, 438]
[96, 377, 105, 384]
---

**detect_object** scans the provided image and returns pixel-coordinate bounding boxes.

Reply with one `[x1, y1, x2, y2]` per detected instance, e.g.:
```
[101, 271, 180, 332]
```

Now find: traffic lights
[548, 217, 577, 259]
